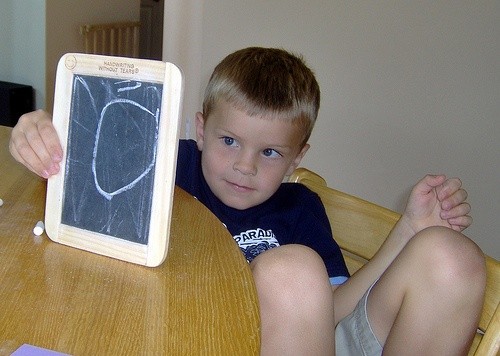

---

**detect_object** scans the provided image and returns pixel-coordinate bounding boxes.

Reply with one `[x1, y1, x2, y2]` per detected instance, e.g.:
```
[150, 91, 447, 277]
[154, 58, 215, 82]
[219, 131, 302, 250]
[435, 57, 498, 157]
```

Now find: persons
[9, 47, 489, 356]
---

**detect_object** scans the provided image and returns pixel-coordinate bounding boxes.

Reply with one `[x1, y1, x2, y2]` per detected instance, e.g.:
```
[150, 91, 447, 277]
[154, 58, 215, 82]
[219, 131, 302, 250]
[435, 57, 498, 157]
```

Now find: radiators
[80, 22, 141, 59]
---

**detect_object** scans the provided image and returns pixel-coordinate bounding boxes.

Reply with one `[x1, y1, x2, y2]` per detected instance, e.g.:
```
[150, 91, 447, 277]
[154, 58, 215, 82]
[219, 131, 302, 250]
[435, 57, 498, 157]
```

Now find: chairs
[286, 167, 500, 356]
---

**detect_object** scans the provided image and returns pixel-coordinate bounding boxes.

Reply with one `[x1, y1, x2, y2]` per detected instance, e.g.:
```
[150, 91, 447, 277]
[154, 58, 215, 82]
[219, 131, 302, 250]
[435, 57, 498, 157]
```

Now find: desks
[0, 125, 262, 355]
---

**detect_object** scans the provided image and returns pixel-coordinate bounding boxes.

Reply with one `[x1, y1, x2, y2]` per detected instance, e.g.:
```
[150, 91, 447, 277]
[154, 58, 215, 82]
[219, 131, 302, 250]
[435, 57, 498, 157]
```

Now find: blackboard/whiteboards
[61, 74, 163, 246]
